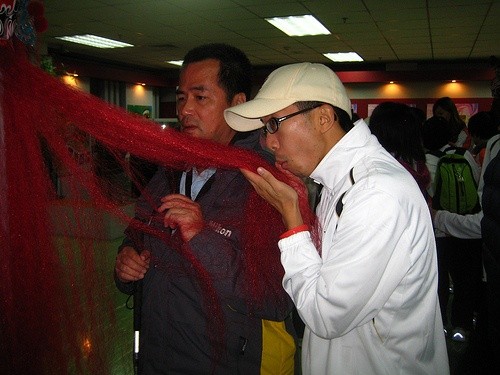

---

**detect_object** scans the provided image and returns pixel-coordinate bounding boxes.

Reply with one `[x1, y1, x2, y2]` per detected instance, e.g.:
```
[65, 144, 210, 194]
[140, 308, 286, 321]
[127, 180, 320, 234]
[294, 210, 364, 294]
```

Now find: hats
[225, 61, 353, 132]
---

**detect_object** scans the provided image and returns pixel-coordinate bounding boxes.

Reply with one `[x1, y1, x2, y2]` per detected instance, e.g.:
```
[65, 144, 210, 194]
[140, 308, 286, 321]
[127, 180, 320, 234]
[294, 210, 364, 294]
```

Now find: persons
[223, 61, 450, 375]
[368, 95, 500, 375]
[113, 42, 299, 374]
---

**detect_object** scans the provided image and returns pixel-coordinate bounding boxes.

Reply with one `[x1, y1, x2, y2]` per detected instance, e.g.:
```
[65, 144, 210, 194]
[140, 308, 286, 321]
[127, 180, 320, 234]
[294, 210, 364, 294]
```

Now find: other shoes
[453, 327, 466, 341]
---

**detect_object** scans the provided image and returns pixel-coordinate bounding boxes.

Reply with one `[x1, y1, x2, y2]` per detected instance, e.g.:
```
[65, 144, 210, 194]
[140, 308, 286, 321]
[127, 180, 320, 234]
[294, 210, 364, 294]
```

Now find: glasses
[261, 102, 338, 132]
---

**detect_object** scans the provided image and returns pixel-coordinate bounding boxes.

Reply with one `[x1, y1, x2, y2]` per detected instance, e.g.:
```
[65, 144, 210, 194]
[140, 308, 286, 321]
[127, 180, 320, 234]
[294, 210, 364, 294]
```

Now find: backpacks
[432, 147, 478, 215]
